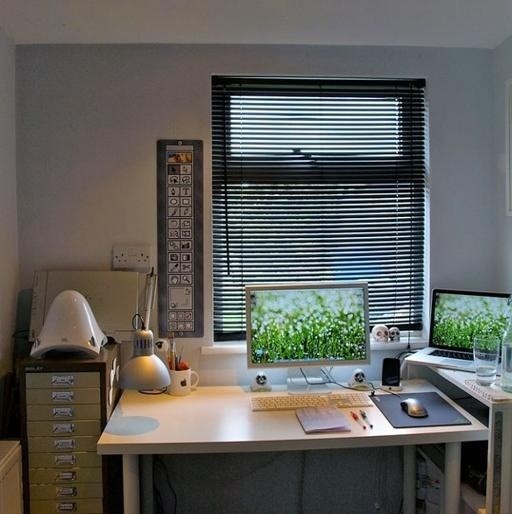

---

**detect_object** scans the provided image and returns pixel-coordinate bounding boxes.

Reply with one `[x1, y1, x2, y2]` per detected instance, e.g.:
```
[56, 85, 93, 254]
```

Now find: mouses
[400, 398, 428, 419]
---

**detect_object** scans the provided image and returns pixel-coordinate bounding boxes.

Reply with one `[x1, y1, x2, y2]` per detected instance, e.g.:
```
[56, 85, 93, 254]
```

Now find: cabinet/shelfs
[16, 341, 121, 514]
[0, 439, 24, 514]
[399, 348, 511, 514]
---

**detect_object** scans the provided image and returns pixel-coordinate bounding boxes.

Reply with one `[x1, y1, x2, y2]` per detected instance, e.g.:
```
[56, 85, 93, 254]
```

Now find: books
[295, 406, 351, 434]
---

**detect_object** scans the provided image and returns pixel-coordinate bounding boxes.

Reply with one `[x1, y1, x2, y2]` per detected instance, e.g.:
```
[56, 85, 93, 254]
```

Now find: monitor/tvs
[244, 281, 371, 393]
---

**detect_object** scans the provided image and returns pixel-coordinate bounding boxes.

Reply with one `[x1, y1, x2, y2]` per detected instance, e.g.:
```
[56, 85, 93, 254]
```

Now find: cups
[168, 369, 200, 395]
[472, 337, 501, 386]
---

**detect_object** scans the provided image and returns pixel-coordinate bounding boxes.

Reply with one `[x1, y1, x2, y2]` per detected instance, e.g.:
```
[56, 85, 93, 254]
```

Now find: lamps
[118, 266, 171, 390]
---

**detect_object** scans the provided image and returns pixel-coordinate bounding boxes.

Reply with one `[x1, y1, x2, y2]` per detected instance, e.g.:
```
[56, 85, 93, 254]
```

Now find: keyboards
[251, 392, 373, 411]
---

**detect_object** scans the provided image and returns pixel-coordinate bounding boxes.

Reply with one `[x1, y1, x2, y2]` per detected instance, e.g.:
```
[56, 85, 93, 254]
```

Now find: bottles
[502, 316, 512, 393]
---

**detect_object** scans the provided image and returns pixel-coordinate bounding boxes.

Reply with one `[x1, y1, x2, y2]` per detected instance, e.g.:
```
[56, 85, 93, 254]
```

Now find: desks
[96, 378, 489, 514]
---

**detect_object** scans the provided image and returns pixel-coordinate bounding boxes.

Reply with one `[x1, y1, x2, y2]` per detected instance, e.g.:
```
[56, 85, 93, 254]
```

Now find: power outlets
[112, 244, 151, 273]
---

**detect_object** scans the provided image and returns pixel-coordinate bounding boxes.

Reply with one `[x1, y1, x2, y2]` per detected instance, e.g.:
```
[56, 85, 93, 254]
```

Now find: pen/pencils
[169, 342, 184, 371]
[350, 410, 373, 429]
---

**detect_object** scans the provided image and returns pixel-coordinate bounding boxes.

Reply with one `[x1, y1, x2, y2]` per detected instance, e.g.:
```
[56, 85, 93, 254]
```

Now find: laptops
[406, 287, 512, 374]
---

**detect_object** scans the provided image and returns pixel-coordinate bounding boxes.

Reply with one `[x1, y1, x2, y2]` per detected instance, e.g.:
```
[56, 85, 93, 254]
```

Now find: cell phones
[382, 358, 400, 385]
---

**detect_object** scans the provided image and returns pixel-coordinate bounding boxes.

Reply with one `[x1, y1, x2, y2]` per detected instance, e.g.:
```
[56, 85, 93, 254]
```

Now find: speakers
[250, 372, 271, 392]
[349, 370, 368, 387]
